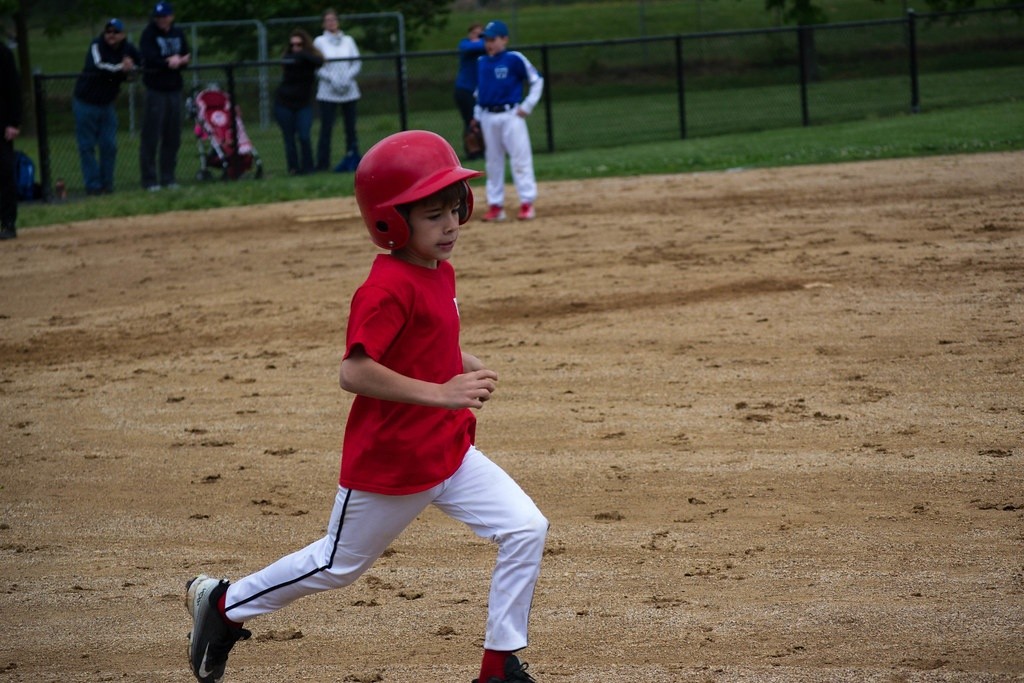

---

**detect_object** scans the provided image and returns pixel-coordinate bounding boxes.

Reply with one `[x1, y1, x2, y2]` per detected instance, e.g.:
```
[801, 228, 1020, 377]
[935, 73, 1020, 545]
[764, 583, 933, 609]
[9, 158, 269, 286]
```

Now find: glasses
[106, 29, 120, 34]
[288, 42, 303, 48]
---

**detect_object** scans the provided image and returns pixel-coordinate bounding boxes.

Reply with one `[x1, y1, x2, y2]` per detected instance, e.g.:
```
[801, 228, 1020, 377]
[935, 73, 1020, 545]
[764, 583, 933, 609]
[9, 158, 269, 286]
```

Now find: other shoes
[0, 223, 16, 239]
[165, 183, 182, 189]
[147, 185, 163, 192]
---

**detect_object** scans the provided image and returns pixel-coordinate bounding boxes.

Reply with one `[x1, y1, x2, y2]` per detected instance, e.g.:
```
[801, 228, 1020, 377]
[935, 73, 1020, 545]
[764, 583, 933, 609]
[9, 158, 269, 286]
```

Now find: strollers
[185, 85, 263, 182]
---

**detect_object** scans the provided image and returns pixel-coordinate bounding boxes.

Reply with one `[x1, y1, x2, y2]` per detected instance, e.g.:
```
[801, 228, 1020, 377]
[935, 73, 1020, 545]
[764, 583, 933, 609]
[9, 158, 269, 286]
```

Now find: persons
[453, 24, 491, 162]
[277, 28, 323, 176]
[310, 7, 362, 173]
[71, 17, 146, 195]
[186, 127, 547, 683]
[474, 20, 545, 221]
[139, 1, 192, 192]
[1, 41, 26, 238]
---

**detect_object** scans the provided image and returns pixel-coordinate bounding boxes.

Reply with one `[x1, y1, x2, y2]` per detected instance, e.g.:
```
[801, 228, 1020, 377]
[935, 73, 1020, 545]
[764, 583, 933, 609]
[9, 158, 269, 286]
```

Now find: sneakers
[482, 204, 506, 222]
[472, 655, 537, 683]
[186, 574, 251, 683]
[518, 203, 536, 220]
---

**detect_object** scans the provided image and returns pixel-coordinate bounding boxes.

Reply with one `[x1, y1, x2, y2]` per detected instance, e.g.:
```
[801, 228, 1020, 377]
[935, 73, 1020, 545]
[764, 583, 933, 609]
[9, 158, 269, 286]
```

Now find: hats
[478, 20, 508, 39]
[105, 18, 124, 28]
[153, 4, 171, 15]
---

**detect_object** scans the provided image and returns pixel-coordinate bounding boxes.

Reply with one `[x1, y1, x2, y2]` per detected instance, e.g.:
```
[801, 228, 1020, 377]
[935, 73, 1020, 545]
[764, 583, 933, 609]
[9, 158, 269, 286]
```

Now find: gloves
[330, 77, 345, 94]
[340, 75, 351, 90]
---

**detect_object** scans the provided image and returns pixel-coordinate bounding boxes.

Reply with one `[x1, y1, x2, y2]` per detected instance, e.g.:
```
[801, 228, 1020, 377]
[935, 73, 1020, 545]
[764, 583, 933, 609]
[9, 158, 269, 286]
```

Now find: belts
[488, 104, 513, 112]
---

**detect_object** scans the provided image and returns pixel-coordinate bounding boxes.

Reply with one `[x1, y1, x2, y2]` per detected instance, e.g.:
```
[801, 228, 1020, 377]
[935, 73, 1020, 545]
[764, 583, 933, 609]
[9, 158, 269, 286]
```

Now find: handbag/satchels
[15, 149, 35, 198]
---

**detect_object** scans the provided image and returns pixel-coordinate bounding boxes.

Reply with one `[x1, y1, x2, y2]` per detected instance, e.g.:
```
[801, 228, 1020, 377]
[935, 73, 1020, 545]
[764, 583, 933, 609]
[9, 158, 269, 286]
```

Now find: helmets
[354, 130, 485, 251]
